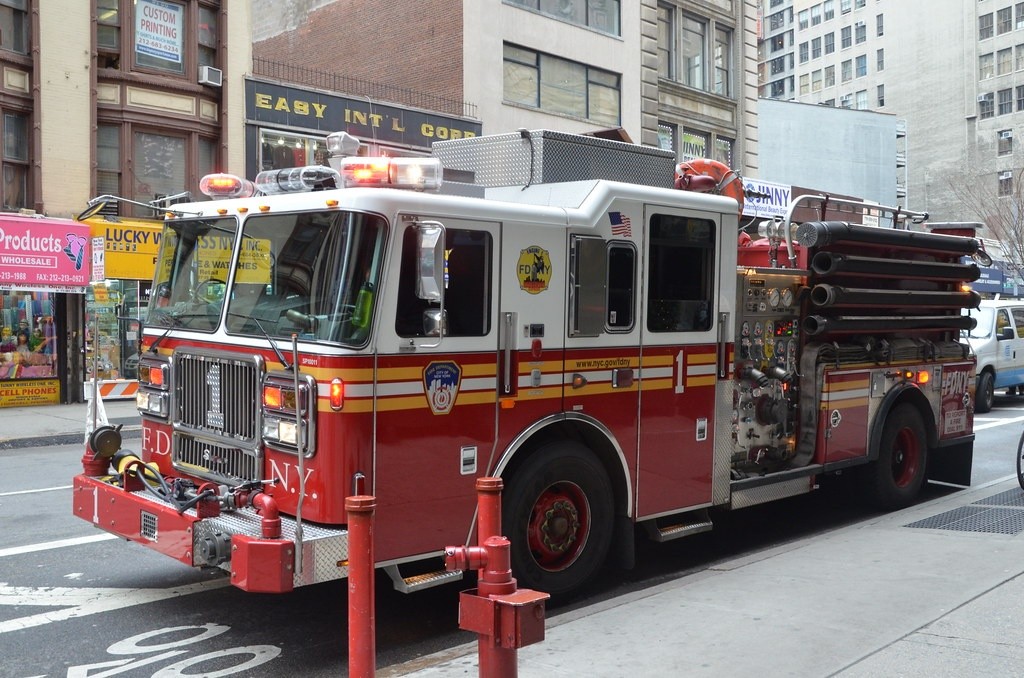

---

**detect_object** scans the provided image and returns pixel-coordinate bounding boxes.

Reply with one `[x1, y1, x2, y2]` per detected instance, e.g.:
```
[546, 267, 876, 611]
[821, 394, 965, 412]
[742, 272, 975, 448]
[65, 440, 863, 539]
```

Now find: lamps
[296, 139, 304, 149]
[278, 136, 285, 144]
[313, 140, 319, 150]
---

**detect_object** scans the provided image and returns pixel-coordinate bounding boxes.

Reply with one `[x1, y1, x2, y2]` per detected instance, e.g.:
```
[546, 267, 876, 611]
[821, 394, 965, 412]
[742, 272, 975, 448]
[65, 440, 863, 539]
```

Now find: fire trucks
[72, 137, 992, 612]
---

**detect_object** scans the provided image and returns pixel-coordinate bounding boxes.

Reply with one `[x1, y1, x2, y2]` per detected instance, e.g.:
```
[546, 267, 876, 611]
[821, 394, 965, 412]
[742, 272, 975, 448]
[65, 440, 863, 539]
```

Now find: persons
[0, 319, 46, 353]
[1006, 384, 1024, 396]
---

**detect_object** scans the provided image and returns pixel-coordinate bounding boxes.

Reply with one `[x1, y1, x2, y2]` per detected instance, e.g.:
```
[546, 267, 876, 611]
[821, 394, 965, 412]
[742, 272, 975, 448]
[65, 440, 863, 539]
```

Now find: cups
[351, 282, 374, 328]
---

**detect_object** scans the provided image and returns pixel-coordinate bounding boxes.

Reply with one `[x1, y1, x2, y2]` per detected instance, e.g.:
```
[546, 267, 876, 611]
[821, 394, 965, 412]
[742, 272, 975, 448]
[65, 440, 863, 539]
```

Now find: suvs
[958, 292, 1023, 414]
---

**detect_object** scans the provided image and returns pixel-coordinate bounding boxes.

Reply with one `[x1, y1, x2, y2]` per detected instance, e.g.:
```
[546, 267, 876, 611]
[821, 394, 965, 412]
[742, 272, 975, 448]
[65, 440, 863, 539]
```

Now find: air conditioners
[977, 95, 987, 102]
[839, 95, 848, 101]
[858, 19, 865, 26]
[1003, 131, 1012, 139]
[1004, 172, 1012, 179]
[197, 65, 223, 87]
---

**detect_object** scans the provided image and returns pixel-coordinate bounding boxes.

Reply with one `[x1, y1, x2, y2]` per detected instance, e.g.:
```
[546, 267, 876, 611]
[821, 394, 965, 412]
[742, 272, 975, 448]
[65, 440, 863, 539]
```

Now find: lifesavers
[675, 158, 746, 220]
